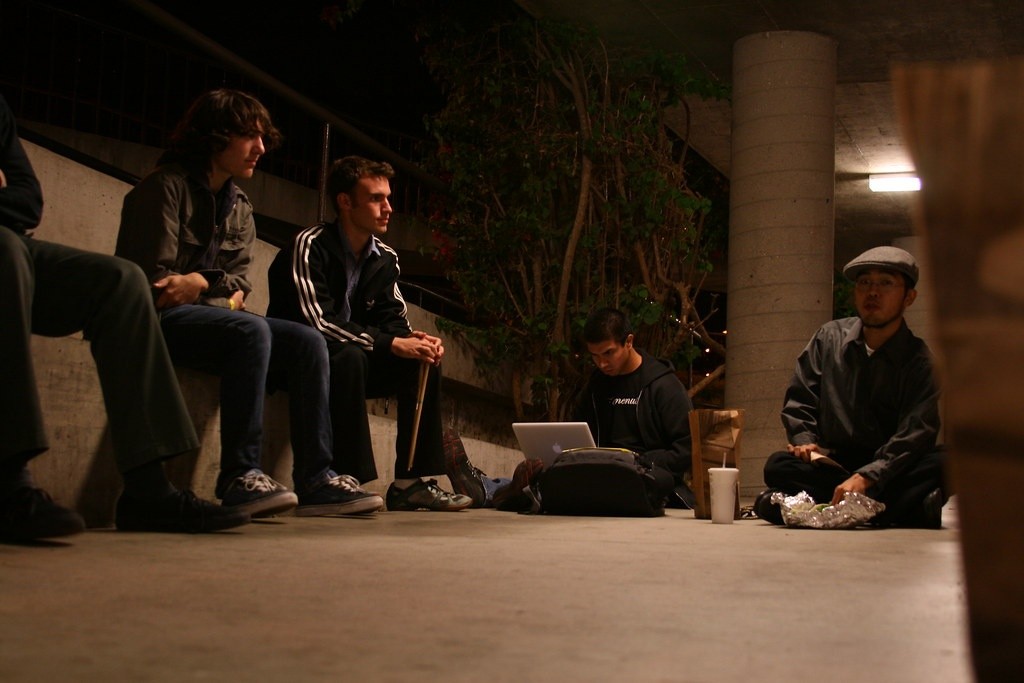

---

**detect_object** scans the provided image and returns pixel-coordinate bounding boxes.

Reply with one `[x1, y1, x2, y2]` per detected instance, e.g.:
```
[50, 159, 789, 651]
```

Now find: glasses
[854, 278, 907, 289]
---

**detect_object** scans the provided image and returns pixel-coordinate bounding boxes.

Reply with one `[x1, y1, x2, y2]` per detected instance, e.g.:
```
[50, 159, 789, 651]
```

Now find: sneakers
[295, 468, 383, 515]
[221, 469, 298, 518]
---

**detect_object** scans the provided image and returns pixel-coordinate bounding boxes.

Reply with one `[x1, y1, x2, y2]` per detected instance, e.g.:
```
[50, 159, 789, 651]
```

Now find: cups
[708, 467, 739, 524]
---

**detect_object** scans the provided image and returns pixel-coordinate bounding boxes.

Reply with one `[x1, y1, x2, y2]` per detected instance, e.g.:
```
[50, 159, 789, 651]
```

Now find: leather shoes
[917, 485, 949, 529]
[752, 488, 819, 523]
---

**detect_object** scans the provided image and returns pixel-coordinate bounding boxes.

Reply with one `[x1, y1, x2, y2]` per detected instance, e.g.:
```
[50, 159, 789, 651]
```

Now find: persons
[115, 89, 384, 524]
[264, 156, 472, 516]
[752, 246, 947, 531]
[439, 307, 695, 515]
[0, 93, 246, 540]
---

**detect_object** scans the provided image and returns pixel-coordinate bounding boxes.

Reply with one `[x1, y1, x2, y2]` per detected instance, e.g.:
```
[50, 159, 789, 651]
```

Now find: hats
[844, 246, 919, 284]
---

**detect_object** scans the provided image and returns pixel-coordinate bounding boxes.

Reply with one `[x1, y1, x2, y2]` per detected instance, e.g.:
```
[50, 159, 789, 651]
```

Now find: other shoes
[115, 465, 252, 531]
[1, 470, 84, 542]
[386, 479, 472, 511]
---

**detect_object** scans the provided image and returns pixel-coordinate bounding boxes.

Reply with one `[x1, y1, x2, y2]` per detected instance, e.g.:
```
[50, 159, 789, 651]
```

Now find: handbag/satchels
[522, 447, 672, 517]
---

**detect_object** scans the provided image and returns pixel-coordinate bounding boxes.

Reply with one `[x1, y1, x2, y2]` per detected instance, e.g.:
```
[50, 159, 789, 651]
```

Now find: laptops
[512, 422, 595, 469]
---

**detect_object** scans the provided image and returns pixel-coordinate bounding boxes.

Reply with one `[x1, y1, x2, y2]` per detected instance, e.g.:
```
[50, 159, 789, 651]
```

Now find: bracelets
[229, 300, 235, 310]
